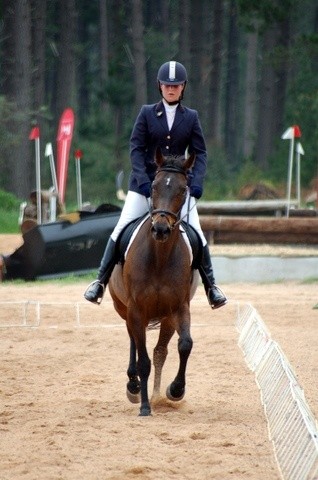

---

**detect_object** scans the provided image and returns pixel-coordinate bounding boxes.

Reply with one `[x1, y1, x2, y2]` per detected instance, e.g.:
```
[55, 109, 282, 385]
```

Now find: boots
[84, 235, 118, 302]
[199, 243, 226, 306]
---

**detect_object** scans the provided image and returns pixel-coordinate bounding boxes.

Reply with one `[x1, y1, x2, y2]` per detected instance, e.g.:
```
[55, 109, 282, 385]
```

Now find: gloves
[140, 182, 152, 198]
[191, 187, 203, 198]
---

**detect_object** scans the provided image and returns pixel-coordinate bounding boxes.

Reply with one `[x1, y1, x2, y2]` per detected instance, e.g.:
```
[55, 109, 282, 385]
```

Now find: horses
[107, 147, 199, 416]
[242, 180, 282, 200]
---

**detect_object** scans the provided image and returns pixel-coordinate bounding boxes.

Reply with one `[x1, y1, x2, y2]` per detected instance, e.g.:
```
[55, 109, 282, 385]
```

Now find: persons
[85, 61, 226, 310]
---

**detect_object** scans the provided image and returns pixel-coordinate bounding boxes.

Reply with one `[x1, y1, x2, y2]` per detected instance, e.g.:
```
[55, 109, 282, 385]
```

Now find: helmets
[157, 61, 188, 85]
